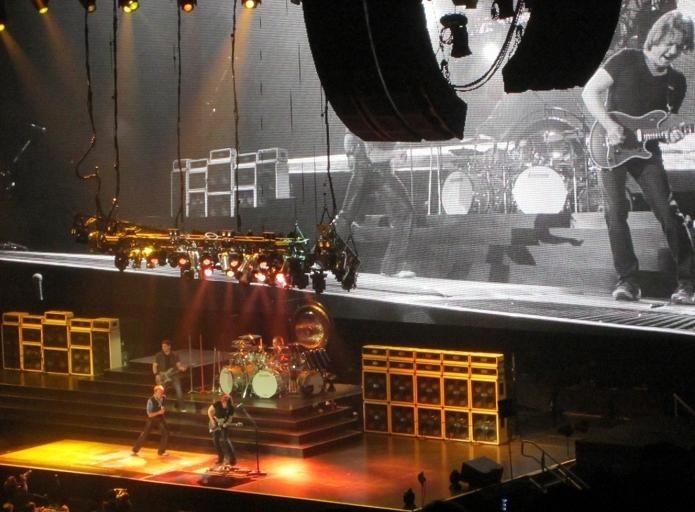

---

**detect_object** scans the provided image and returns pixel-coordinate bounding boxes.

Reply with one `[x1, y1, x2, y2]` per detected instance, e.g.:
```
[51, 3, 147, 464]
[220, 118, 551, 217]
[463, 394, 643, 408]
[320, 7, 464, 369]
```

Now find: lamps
[115, 246, 326, 296]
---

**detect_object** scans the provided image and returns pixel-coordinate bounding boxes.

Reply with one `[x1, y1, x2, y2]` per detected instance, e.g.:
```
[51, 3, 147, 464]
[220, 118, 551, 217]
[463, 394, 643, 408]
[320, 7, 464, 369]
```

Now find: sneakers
[210, 463, 237, 472]
[131, 450, 169, 457]
[669, 287, 695, 305]
[612, 282, 642, 302]
[380, 269, 416, 279]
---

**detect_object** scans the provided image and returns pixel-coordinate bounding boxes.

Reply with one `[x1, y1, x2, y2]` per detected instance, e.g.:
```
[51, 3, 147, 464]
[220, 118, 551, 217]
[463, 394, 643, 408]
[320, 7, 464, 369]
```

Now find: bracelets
[153, 372, 159, 376]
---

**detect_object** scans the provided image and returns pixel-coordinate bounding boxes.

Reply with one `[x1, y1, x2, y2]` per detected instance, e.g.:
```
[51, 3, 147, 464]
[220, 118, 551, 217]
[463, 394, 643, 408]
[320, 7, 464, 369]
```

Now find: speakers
[299, 0, 467, 143]
[500, 0, 622, 94]
[460, 456, 504, 488]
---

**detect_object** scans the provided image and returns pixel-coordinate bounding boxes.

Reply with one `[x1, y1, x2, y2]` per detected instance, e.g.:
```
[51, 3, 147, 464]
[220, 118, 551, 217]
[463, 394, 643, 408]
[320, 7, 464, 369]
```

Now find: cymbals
[289, 342, 299, 346]
[450, 150, 484, 156]
[232, 335, 262, 349]
[269, 346, 288, 350]
[451, 159, 470, 167]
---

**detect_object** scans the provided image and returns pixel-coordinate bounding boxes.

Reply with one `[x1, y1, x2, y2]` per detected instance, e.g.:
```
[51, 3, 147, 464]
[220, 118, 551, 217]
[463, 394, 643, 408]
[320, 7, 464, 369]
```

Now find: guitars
[155, 368, 181, 385]
[209, 416, 243, 433]
[589, 111, 695, 169]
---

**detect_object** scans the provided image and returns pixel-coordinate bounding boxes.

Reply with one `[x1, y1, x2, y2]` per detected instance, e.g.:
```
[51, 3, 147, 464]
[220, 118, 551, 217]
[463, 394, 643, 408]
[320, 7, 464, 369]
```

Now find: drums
[252, 369, 283, 399]
[512, 166, 568, 214]
[297, 371, 322, 394]
[219, 365, 248, 395]
[441, 171, 491, 215]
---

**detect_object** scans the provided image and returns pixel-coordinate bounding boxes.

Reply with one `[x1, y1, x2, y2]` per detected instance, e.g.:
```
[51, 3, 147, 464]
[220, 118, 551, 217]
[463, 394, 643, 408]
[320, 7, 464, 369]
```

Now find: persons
[2, 474, 70, 512]
[207, 394, 236, 465]
[152, 341, 187, 413]
[129, 385, 169, 456]
[310, 124, 417, 279]
[581, 8, 695, 305]
